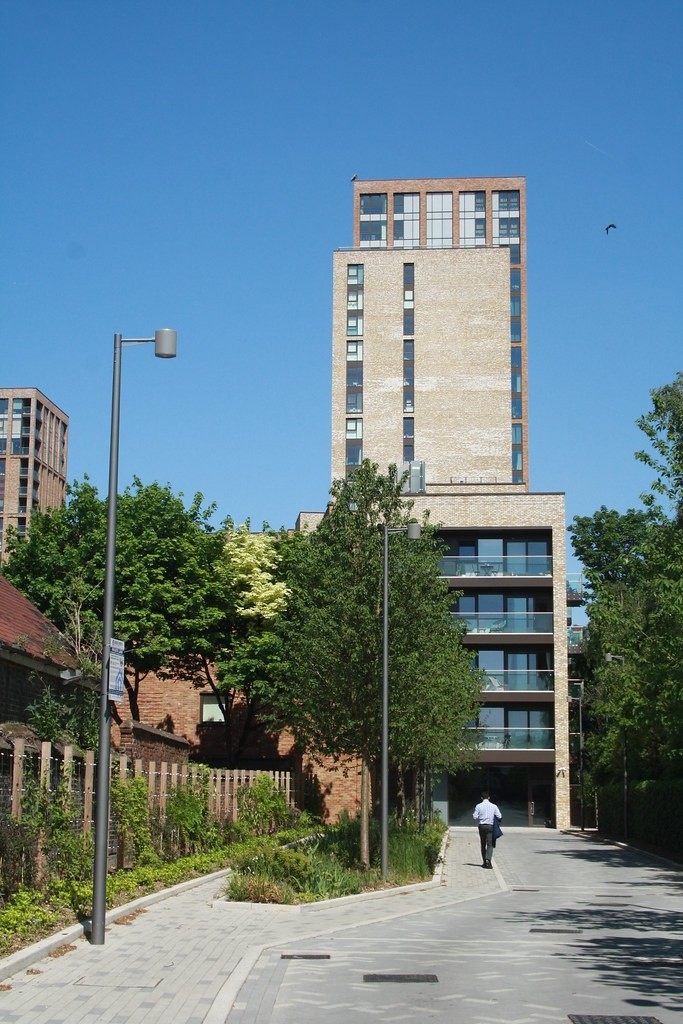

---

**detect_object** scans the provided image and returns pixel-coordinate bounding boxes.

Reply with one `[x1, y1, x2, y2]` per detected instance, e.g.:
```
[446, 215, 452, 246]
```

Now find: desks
[484, 736, 499, 748]
[480, 565, 493, 575]
[479, 628, 486, 633]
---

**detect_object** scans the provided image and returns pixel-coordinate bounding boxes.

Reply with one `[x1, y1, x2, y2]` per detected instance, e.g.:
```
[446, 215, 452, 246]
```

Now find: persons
[473, 792, 502, 868]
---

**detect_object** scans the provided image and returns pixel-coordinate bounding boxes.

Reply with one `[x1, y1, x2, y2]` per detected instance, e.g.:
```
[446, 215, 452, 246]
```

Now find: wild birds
[604, 224, 616, 234]
[351, 174, 357, 182]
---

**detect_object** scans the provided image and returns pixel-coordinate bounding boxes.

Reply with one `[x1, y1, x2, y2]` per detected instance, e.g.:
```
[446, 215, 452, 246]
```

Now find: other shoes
[484, 860, 492, 869]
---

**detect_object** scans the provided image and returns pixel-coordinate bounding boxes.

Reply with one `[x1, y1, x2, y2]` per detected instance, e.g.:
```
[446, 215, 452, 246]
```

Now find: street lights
[380, 523, 421, 876]
[567, 696, 585, 832]
[604, 652, 629, 838]
[90, 328, 177, 945]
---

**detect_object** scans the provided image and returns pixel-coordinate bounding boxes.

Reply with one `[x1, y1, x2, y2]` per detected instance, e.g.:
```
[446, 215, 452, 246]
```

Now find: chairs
[491, 620, 507, 632]
[488, 676, 508, 690]
[492, 564, 500, 576]
[471, 564, 480, 575]
